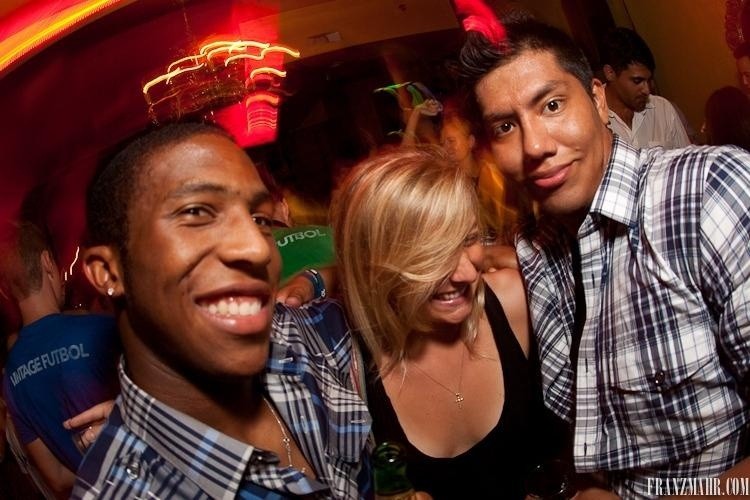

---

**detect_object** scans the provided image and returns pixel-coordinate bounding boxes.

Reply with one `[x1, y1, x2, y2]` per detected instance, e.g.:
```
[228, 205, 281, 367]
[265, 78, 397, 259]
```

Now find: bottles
[370, 440, 415, 500]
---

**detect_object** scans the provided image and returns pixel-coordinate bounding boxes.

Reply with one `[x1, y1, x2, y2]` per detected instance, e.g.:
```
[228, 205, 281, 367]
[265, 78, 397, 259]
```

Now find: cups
[69, 417, 110, 459]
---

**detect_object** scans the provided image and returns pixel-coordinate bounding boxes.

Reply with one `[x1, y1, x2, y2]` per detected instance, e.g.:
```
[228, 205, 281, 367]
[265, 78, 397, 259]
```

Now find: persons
[0, 8, 750, 500]
